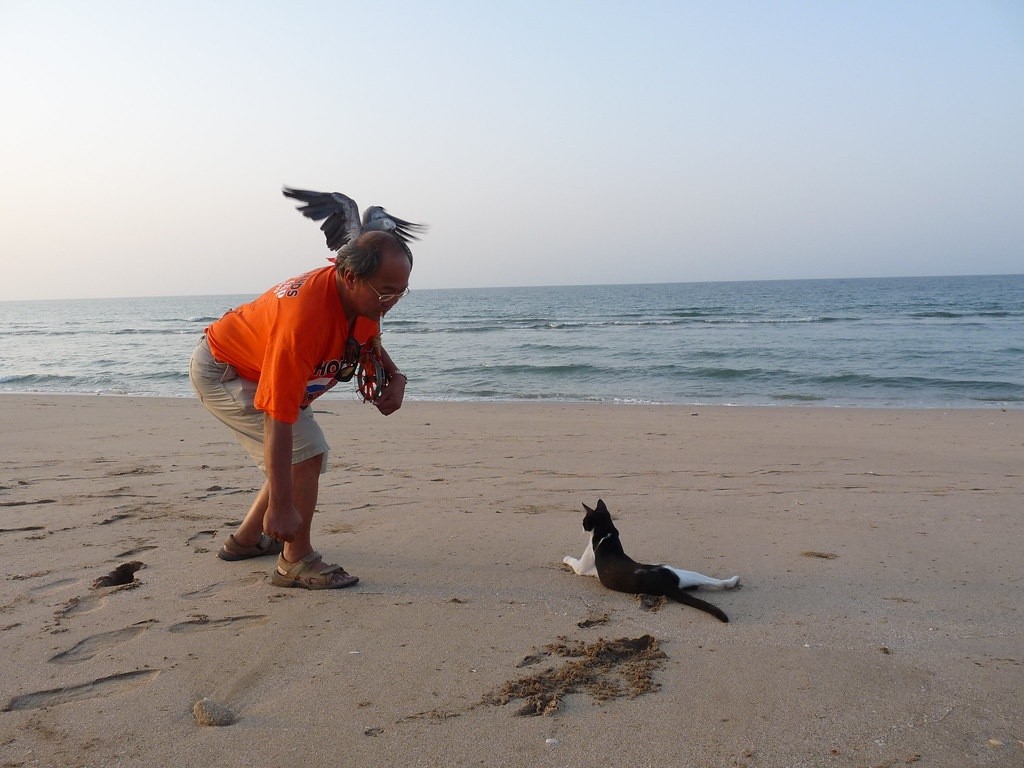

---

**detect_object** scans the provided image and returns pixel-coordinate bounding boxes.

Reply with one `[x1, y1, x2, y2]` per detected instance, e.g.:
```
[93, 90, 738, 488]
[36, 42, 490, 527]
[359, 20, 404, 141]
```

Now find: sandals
[218, 534, 278, 560]
[272, 552, 358, 589]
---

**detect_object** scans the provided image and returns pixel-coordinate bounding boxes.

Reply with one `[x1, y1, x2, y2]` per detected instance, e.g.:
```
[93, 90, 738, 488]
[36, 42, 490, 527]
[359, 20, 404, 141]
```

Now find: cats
[563, 498, 741, 625]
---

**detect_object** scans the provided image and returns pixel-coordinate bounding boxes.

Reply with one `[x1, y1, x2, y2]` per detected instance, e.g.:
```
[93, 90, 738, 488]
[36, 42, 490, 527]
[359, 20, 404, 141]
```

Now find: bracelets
[387, 368, 408, 385]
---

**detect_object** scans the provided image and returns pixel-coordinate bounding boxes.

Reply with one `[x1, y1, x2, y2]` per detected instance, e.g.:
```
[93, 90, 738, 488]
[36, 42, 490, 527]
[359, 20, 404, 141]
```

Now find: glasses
[335, 336, 360, 382]
[361, 279, 411, 303]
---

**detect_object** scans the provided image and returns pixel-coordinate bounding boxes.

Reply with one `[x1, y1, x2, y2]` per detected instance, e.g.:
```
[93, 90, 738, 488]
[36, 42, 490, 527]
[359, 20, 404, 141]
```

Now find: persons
[188, 229, 411, 589]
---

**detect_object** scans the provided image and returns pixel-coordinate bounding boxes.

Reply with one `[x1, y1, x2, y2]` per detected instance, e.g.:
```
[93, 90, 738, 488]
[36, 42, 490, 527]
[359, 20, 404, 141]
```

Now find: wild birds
[282, 187, 426, 251]
